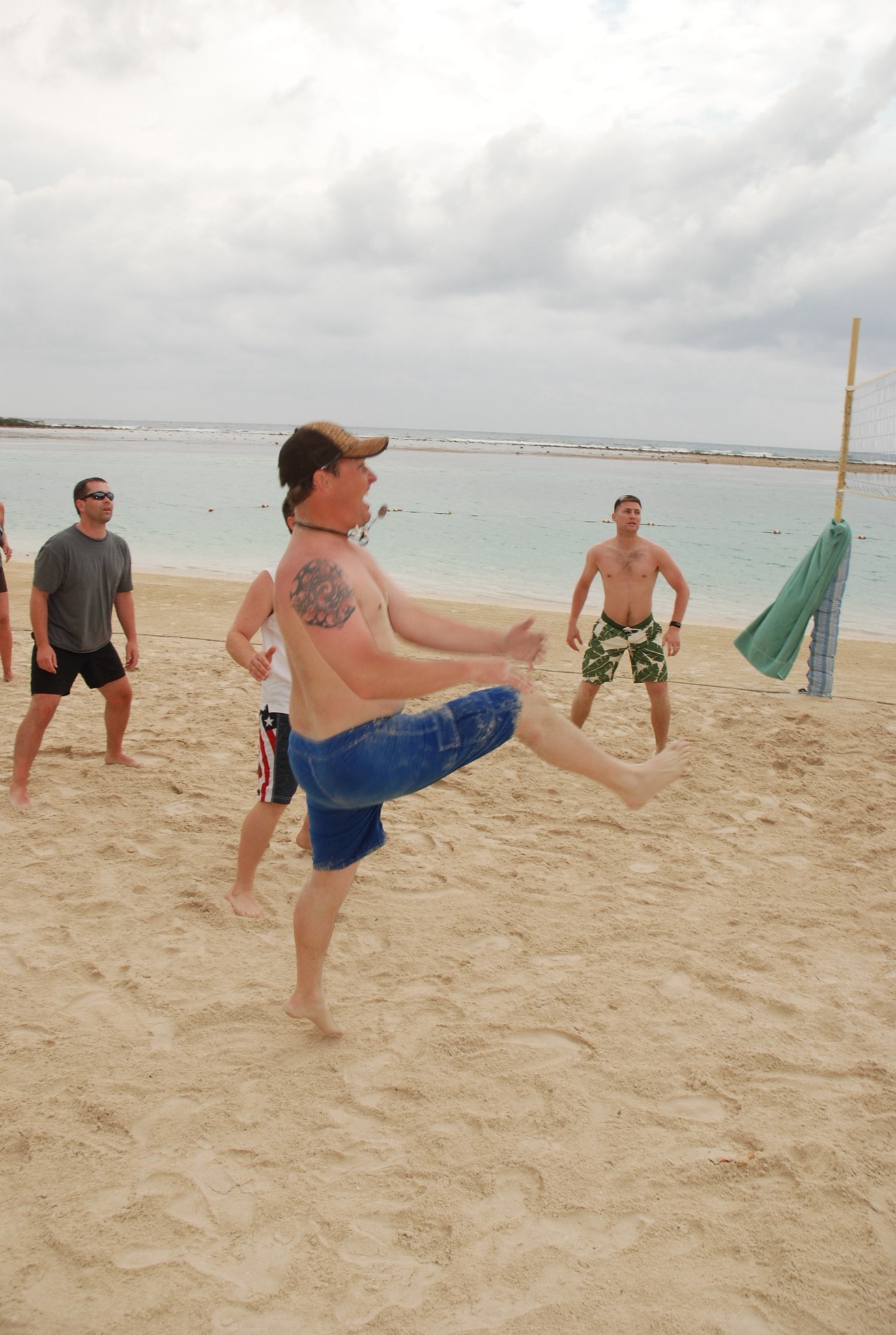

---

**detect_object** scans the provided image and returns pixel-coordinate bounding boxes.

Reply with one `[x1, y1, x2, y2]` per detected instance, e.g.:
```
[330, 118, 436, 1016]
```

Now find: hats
[277, 422, 388, 487]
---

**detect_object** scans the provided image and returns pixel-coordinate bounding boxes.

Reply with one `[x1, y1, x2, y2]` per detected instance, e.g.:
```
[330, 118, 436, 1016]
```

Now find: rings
[677, 647, 679, 648]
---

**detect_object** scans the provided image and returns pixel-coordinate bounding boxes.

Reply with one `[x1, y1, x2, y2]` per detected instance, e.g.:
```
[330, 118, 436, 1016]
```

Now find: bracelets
[670, 621, 681, 628]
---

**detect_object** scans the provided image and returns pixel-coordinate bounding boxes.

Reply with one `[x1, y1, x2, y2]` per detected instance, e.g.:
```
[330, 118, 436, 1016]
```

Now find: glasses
[79, 492, 114, 500]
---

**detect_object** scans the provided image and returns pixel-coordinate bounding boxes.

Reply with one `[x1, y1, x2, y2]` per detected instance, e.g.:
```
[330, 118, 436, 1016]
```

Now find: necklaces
[293, 519, 347, 539]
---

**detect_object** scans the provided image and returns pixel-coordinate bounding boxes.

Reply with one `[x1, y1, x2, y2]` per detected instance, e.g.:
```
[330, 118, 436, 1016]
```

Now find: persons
[221, 494, 313, 917]
[273, 423, 693, 1040]
[7, 477, 145, 810]
[565, 495, 690, 755]
[0, 503, 16, 682]
